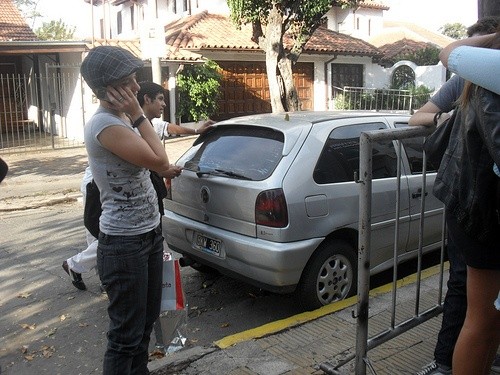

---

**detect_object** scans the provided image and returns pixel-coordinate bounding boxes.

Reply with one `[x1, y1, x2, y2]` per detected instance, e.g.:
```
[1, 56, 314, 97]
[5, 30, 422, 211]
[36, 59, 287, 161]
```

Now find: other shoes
[62, 260, 87, 291]
[438, 365, 452, 375]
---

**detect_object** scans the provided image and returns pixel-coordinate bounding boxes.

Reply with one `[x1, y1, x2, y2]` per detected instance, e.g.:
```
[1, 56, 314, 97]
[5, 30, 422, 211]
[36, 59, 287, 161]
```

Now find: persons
[150, 118, 218, 142]
[79, 43, 171, 375]
[60, 80, 166, 289]
[407, 14, 500, 375]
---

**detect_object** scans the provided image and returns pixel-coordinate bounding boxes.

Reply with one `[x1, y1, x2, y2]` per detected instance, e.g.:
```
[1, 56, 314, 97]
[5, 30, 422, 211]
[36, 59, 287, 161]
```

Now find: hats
[81, 46, 144, 89]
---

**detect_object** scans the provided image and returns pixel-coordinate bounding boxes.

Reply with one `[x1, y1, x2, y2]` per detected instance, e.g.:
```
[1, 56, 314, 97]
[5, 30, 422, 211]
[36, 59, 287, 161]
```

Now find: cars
[161, 109, 450, 312]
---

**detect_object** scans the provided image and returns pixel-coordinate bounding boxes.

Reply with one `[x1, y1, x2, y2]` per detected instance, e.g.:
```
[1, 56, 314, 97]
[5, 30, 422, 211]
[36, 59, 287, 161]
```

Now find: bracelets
[131, 114, 146, 128]
[434, 111, 447, 125]
[195, 128, 198, 134]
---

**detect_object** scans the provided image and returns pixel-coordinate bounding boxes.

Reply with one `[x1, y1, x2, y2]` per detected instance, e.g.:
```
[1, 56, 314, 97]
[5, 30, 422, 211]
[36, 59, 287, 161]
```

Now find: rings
[119, 97, 124, 102]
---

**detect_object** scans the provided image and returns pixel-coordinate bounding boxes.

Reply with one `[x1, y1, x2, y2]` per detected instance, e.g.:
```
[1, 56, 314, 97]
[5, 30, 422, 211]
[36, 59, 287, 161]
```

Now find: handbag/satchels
[422, 106, 459, 172]
[159, 258, 187, 311]
[84, 167, 166, 240]
